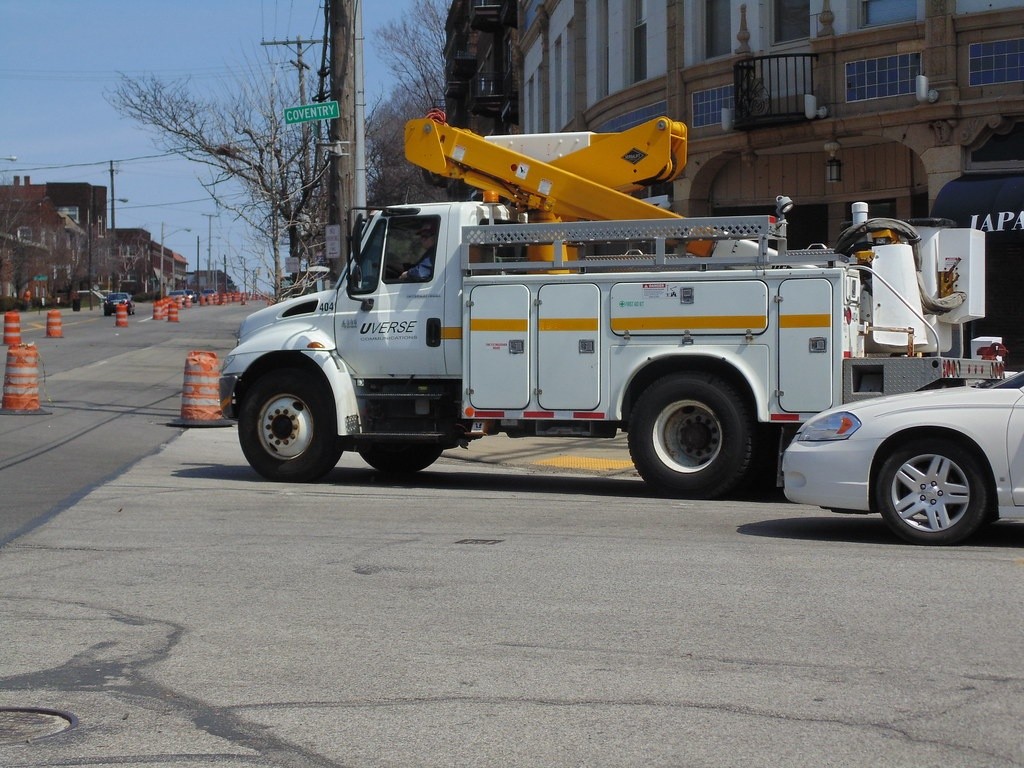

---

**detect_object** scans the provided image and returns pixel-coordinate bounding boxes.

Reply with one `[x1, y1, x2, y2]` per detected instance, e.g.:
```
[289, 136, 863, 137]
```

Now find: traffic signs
[284, 101, 340, 126]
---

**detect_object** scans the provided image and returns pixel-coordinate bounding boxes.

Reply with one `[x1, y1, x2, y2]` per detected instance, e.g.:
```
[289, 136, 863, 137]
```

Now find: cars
[168, 290, 188, 304]
[103, 293, 136, 317]
[201, 289, 216, 301]
[182, 289, 197, 303]
[779, 367, 1024, 547]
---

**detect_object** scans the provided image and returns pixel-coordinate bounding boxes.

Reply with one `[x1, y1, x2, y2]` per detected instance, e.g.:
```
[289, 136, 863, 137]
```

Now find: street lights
[160, 221, 191, 300]
[87, 199, 128, 311]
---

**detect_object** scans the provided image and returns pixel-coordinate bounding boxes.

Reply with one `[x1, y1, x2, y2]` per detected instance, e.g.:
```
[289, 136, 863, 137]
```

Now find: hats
[415, 224, 437, 235]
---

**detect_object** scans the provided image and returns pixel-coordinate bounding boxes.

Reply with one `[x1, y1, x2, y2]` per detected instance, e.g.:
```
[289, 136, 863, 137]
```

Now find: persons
[399, 230, 437, 279]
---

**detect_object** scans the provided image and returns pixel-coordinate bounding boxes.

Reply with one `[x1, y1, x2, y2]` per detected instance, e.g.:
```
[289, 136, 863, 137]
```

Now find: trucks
[215, 110, 990, 501]
